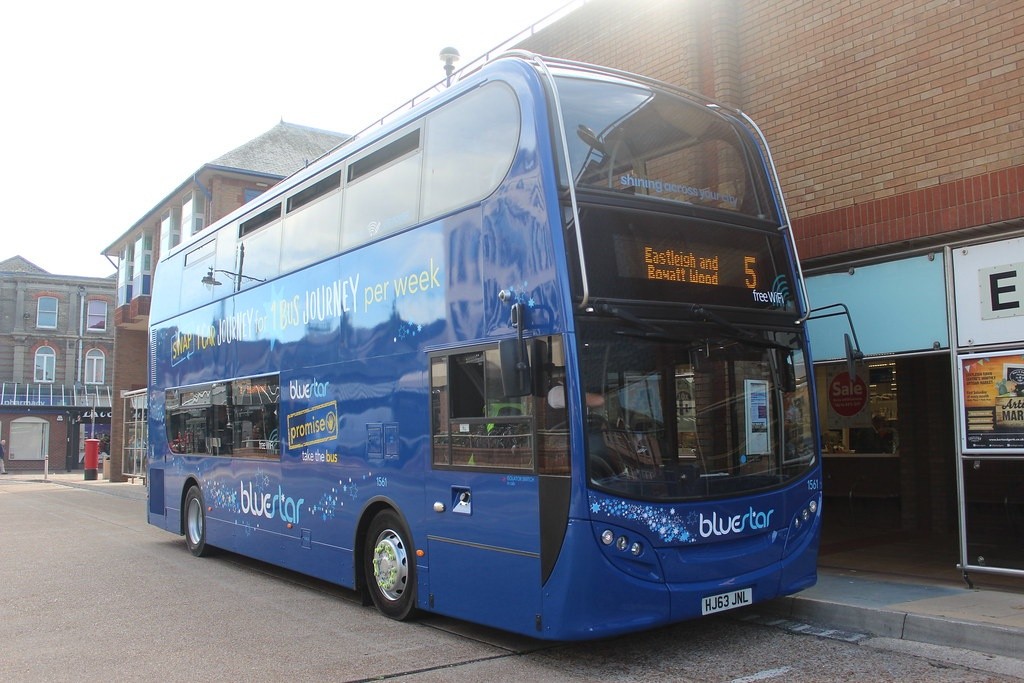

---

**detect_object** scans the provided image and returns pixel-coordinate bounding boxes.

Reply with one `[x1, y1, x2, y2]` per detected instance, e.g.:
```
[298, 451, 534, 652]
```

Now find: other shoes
[2, 472, 8, 474]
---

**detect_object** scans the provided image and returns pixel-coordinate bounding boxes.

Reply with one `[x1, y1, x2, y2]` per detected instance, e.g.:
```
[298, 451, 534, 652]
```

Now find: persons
[0, 440, 8, 474]
[535, 378, 606, 428]
[856, 415, 895, 453]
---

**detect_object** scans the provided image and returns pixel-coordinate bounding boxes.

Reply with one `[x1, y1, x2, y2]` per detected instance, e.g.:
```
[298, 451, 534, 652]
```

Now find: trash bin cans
[102, 455, 111, 480]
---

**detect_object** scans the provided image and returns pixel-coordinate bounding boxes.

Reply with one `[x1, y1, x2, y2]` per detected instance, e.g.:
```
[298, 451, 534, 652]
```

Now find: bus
[145, 48, 867, 641]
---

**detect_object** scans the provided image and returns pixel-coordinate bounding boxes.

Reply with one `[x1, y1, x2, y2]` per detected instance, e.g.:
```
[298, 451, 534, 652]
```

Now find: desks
[822, 452, 902, 510]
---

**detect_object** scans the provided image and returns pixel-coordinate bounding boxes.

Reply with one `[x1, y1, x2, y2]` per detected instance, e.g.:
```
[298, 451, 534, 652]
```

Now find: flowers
[99, 435, 111, 456]
